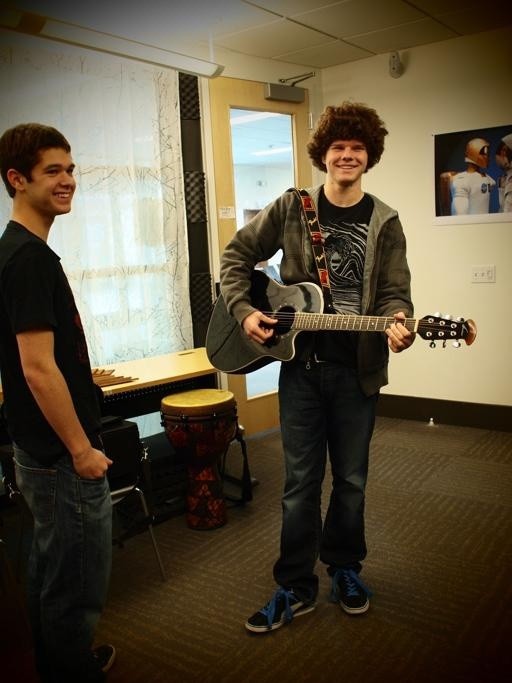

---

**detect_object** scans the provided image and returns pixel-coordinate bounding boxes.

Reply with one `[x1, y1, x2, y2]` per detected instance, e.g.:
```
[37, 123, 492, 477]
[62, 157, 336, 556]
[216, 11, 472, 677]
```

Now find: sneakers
[329, 568, 371, 614]
[245, 588, 314, 632]
[92, 644, 117, 672]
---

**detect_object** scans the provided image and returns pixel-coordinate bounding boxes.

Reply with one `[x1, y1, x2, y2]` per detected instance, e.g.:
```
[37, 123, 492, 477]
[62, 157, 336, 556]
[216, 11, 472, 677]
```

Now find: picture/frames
[431, 123, 512, 227]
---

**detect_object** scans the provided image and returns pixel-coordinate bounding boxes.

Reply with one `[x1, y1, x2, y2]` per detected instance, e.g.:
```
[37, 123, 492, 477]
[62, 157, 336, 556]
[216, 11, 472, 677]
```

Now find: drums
[159, 386, 240, 531]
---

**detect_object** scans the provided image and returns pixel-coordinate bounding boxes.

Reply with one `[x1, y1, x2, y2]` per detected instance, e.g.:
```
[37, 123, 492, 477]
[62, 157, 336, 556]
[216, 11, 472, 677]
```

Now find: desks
[91, 346, 253, 511]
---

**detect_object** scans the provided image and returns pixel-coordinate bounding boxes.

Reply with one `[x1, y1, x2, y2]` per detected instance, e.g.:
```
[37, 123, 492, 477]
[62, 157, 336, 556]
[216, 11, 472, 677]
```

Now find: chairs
[93, 383, 167, 585]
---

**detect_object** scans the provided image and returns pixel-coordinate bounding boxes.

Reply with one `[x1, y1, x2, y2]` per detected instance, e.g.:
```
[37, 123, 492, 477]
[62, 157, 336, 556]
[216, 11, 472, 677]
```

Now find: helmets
[465, 138, 490, 168]
[496, 134, 512, 167]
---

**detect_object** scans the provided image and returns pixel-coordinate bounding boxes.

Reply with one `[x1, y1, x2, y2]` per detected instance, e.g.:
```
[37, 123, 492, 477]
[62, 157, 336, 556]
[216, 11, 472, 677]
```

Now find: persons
[495, 133, 512, 214]
[218, 99, 417, 637]
[0, 121, 119, 682]
[448, 138, 496, 216]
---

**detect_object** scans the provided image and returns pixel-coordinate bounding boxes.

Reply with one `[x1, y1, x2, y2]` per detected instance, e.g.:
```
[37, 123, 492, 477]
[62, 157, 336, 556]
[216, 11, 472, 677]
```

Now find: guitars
[204, 268, 479, 376]
